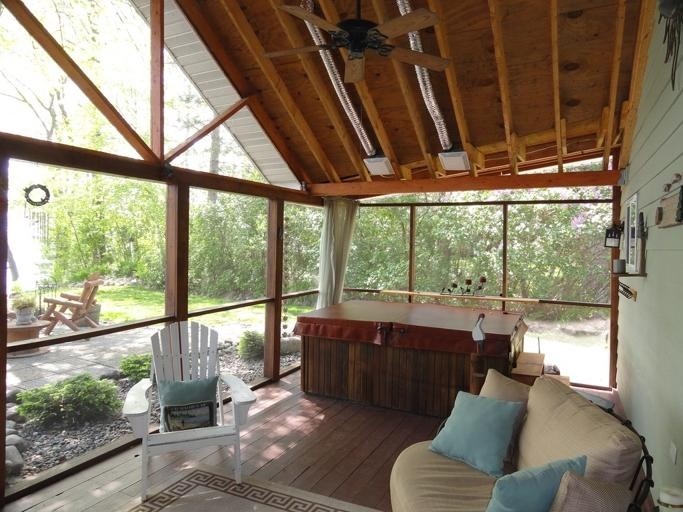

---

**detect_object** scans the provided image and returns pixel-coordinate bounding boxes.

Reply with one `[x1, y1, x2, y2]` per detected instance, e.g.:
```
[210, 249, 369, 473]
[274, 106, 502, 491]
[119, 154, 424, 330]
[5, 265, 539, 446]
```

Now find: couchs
[389, 368, 655, 511]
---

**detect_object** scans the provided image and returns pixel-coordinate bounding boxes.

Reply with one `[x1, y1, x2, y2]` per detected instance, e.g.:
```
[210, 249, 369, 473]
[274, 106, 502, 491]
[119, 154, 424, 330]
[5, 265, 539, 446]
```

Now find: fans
[263, 1, 452, 85]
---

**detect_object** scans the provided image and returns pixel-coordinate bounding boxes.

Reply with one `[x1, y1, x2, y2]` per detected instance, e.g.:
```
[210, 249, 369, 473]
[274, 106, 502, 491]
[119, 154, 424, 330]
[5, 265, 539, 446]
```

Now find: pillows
[485, 452, 589, 511]
[430, 388, 524, 478]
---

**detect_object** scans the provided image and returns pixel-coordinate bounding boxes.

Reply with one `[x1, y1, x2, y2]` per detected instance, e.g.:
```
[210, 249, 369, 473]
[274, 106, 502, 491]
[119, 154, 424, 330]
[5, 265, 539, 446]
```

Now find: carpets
[126, 462, 380, 512]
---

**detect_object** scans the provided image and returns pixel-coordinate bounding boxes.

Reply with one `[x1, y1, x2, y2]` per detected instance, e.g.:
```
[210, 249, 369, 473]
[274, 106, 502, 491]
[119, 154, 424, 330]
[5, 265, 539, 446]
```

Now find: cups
[612, 259, 626, 274]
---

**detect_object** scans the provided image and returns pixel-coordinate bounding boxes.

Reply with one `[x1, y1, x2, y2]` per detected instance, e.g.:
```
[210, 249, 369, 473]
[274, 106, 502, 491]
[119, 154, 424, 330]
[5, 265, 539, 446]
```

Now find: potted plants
[11, 287, 37, 325]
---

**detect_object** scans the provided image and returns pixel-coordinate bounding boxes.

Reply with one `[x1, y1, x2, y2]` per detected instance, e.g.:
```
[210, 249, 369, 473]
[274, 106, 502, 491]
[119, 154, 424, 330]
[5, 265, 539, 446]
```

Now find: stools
[511, 352, 545, 388]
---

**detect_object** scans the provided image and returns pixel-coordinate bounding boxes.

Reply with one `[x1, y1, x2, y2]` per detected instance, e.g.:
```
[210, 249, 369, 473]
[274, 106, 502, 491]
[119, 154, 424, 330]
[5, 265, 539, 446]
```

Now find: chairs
[41, 278, 103, 343]
[120, 319, 258, 503]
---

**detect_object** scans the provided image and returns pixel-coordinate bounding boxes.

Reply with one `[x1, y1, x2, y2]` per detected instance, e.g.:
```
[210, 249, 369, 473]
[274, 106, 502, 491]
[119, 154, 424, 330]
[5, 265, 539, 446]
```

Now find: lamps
[604, 221, 626, 248]
